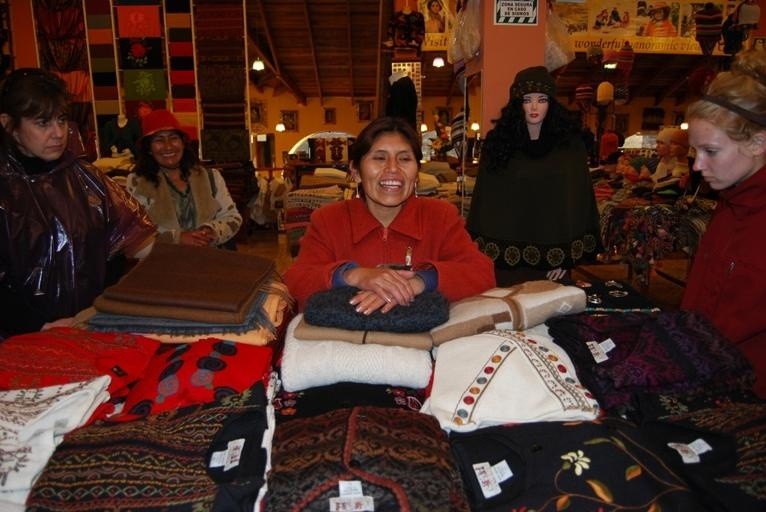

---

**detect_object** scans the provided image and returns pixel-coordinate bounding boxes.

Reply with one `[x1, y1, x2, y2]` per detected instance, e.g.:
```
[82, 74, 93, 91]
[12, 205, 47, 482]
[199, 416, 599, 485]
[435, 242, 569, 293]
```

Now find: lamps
[471, 119, 481, 146]
[252, 56, 266, 72]
[108, 0, 128, 129]
[591, 81, 614, 168]
[420, 122, 428, 134]
[273, 114, 287, 132]
[433, 51, 446, 67]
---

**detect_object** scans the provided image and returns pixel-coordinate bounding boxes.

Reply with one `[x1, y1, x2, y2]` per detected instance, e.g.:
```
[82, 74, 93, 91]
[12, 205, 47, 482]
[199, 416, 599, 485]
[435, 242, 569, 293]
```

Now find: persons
[677, 69, 765, 412]
[593, 8, 609, 30]
[646, 127, 683, 182]
[643, 2, 677, 38]
[280, 117, 500, 317]
[1, 65, 155, 343]
[422, 0, 446, 33]
[607, 8, 619, 27]
[620, 12, 630, 28]
[385, 71, 419, 134]
[459, 64, 609, 293]
[385, 7, 425, 49]
[668, 129, 689, 182]
[121, 108, 246, 255]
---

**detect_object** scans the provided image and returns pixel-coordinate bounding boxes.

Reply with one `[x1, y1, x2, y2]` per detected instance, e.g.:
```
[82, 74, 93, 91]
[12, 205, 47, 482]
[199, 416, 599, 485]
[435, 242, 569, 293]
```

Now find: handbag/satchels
[220, 234, 239, 250]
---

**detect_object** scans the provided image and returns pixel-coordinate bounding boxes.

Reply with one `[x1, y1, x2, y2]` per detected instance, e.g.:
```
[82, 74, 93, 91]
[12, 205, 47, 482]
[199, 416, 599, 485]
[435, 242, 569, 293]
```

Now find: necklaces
[163, 172, 191, 198]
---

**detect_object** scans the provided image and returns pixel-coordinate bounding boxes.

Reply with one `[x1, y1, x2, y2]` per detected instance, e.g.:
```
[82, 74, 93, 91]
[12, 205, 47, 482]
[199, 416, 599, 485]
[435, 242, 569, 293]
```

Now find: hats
[656, 127, 680, 144]
[599, 131, 619, 161]
[643, 157, 659, 175]
[510, 64, 556, 99]
[669, 130, 688, 148]
[648, 0, 671, 18]
[139, 109, 185, 138]
[586, 45, 603, 62]
[737, 5, 761, 27]
[596, 81, 615, 105]
[574, 84, 595, 105]
[602, 49, 620, 65]
[694, 2, 723, 42]
[618, 40, 635, 70]
[629, 155, 644, 174]
[722, 13, 745, 55]
